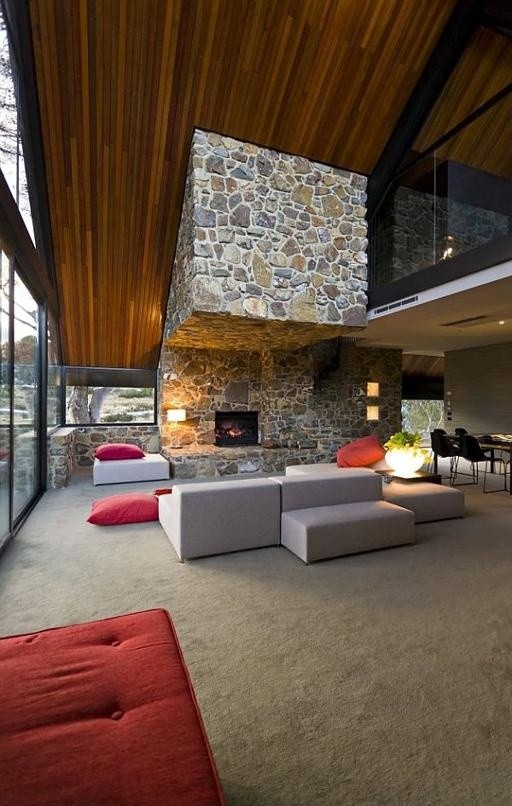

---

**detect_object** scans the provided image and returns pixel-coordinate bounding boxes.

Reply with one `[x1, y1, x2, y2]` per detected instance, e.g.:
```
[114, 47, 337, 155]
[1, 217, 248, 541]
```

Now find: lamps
[166, 408, 189, 450]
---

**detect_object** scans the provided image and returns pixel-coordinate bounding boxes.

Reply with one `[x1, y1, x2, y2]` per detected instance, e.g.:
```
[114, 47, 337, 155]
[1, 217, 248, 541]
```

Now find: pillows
[86, 492, 159, 528]
[93, 442, 146, 461]
[335, 434, 382, 469]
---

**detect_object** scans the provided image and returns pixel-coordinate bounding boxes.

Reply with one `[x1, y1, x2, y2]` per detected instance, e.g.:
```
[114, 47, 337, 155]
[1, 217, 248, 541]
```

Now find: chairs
[429, 427, 512, 498]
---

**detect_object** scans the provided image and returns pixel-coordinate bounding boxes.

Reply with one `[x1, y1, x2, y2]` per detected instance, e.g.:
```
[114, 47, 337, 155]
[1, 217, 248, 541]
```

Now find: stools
[383, 481, 464, 520]
[92, 452, 171, 485]
[279, 498, 414, 562]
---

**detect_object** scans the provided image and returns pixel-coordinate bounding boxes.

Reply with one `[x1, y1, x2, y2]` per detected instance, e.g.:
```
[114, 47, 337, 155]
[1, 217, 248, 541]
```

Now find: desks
[376, 467, 441, 484]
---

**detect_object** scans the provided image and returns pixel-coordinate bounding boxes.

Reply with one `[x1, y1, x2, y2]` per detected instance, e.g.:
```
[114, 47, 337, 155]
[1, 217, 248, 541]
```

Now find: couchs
[157, 463, 382, 560]
